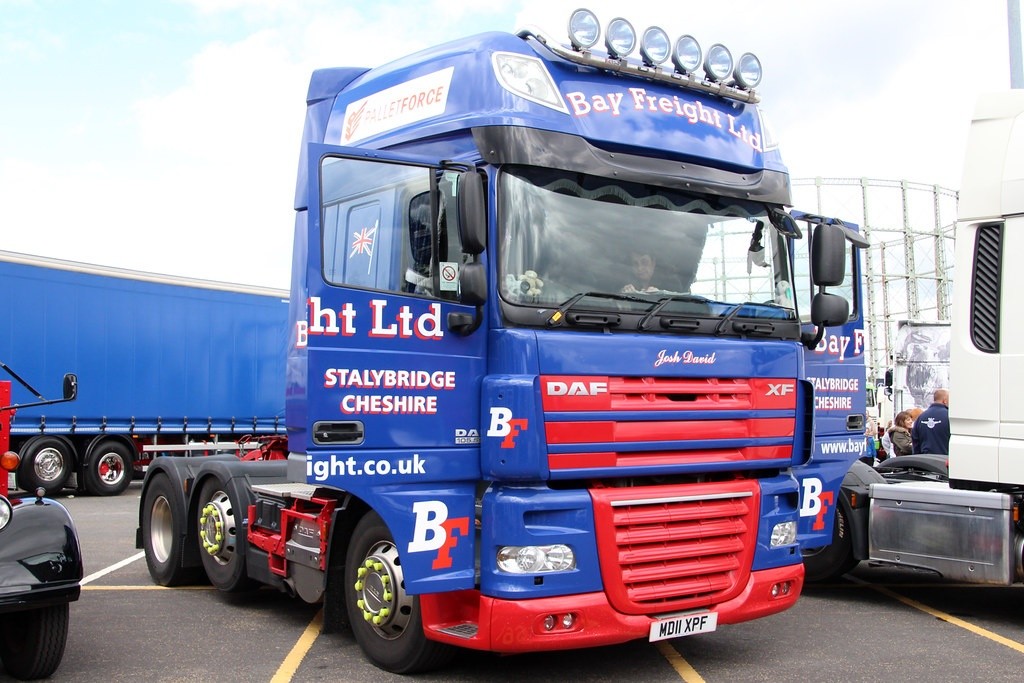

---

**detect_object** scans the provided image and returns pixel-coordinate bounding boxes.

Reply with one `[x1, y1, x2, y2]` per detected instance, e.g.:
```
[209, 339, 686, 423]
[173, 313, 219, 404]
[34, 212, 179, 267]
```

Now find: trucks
[1, 361, 85, 683]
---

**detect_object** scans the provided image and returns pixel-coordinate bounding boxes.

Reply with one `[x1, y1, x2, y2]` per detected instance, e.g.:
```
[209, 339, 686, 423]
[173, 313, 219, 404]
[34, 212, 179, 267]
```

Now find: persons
[858, 389, 951, 466]
[619, 242, 683, 296]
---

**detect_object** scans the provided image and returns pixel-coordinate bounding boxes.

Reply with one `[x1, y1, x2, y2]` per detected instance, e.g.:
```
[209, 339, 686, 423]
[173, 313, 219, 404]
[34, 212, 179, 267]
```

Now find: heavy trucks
[0, 248, 289, 499]
[129, 6, 872, 678]
[793, 86, 1023, 621]
